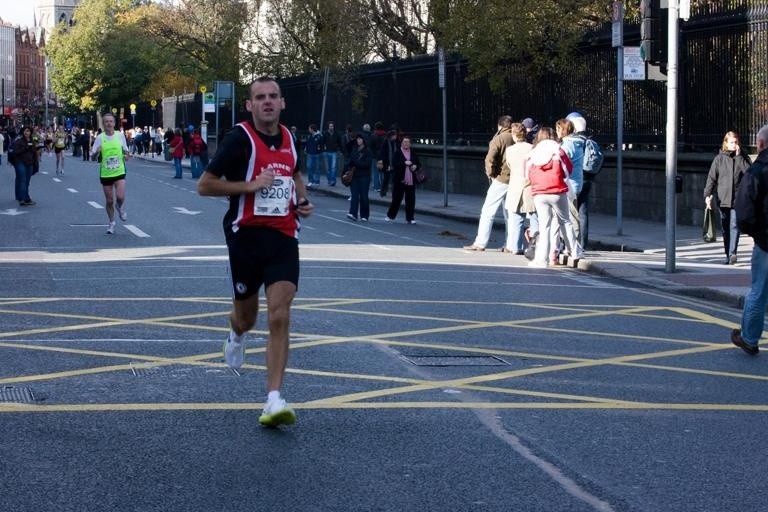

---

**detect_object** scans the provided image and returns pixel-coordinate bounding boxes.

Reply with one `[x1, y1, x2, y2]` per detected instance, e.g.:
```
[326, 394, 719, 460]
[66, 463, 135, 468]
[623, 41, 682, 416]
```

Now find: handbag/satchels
[168, 147, 175, 153]
[191, 139, 208, 155]
[341, 170, 354, 187]
[702, 206, 717, 243]
[409, 164, 430, 184]
[32, 159, 39, 175]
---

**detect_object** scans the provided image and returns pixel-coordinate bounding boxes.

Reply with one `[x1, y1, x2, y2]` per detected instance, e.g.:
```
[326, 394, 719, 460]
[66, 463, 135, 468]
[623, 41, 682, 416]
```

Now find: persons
[704, 132, 752, 265]
[7, 126, 40, 205]
[290, 121, 420, 224]
[119, 126, 209, 180]
[197, 77, 315, 426]
[730, 125, 768, 357]
[90, 113, 129, 235]
[2, 122, 102, 175]
[463, 113, 593, 266]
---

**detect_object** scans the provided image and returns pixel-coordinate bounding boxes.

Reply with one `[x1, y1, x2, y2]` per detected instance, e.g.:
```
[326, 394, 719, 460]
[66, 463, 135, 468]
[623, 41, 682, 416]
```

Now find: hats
[522, 117, 537, 128]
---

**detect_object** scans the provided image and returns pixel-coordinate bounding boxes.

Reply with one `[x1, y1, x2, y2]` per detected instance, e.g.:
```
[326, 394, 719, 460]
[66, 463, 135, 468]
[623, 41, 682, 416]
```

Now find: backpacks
[7, 140, 17, 165]
[580, 137, 604, 175]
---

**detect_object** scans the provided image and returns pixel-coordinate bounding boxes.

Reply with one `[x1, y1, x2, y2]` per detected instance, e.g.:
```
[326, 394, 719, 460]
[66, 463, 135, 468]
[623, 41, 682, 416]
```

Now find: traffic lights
[638, 0, 659, 65]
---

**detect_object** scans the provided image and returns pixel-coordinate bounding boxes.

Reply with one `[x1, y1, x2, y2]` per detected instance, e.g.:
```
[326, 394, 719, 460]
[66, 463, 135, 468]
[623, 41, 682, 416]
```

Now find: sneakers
[307, 182, 320, 187]
[56, 169, 64, 177]
[222, 324, 245, 370]
[405, 219, 416, 225]
[371, 186, 382, 191]
[114, 202, 128, 221]
[385, 217, 398, 224]
[730, 328, 759, 355]
[724, 253, 738, 265]
[18, 198, 36, 206]
[329, 181, 336, 186]
[462, 244, 486, 252]
[258, 397, 297, 428]
[346, 213, 358, 221]
[362, 218, 368, 222]
[106, 220, 117, 235]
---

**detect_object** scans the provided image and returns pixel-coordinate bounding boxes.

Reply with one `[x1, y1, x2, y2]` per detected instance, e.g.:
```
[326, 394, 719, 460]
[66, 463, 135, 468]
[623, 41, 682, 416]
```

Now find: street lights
[5, 96, 11, 127]
[199, 85, 209, 144]
[130, 103, 137, 129]
[112, 108, 118, 129]
[150, 99, 158, 157]
[44, 61, 50, 129]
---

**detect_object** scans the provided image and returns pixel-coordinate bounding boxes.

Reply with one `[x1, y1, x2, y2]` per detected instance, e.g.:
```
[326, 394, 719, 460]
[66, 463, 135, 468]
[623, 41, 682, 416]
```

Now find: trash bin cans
[164, 144, 173, 161]
